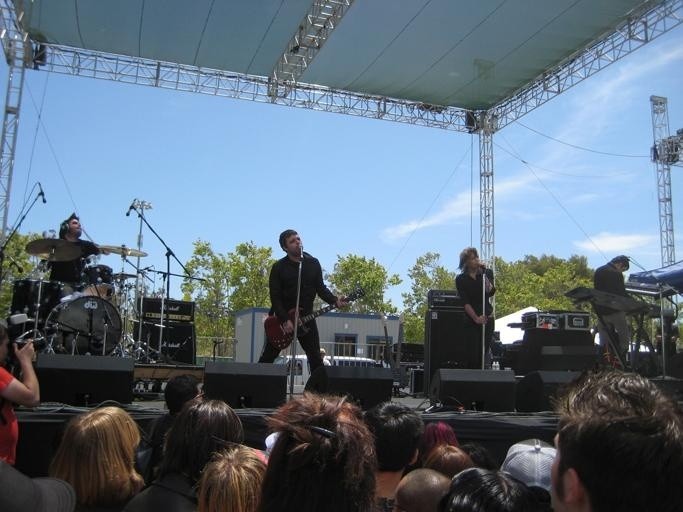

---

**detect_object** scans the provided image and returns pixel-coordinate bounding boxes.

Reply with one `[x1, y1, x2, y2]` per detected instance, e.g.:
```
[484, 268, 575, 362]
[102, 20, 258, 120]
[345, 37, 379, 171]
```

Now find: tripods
[110, 211, 207, 365]
[13, 265, 56, 353]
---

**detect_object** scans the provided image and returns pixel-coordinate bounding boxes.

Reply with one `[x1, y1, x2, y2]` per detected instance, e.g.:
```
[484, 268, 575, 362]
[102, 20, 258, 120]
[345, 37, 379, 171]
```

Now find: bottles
[492, 361, 499, 370]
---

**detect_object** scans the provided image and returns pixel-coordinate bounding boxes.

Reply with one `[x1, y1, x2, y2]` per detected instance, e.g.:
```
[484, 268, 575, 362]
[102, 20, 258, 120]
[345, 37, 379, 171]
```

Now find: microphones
[39, 183, 46, 203]
[126, 199, 136, 216]
[10, 256, 23, 273]
[300, 246, 303, 259]
[479, 263, 486, 272]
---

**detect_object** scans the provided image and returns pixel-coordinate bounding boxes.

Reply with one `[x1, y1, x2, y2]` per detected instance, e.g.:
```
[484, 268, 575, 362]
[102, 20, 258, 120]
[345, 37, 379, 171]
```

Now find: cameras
[8, 337, 48, 363]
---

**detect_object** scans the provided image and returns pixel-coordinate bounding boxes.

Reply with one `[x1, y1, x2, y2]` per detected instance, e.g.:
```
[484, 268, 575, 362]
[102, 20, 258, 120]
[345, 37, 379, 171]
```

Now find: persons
[455, 246, 497, 361]
[255, 229, 348, 376]
[593, 255, 631, 363]
[38, 212, 86, 286]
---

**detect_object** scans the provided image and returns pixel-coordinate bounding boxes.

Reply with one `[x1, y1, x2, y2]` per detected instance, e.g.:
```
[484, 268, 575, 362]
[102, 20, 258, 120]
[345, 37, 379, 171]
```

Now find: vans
[275, 356, 379, 388]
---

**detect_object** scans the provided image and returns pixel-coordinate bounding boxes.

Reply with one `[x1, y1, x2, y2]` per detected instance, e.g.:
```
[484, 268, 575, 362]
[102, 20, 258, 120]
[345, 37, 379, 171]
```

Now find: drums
[44, 293, 122, 356]
[74, 265, 114, 299]
[11, 279, 63, 352]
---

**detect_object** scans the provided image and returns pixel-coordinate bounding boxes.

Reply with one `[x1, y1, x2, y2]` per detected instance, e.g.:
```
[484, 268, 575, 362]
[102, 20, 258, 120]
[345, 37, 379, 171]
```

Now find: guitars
[264, 286, 364, 350]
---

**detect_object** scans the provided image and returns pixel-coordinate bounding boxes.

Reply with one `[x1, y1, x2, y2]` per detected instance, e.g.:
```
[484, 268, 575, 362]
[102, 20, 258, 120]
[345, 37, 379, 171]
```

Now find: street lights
[130, 198, 153, 322]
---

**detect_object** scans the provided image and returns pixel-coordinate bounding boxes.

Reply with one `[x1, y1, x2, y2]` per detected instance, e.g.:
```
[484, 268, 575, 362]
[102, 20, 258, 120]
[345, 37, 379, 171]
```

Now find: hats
[502, 439, 560, 492]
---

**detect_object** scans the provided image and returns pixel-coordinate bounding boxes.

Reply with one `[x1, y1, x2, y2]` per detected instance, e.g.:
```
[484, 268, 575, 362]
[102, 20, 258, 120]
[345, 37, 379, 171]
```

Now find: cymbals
[101, 245, 148, 258]
[111, 274, 137, 280]
[25, 238, 81, 261]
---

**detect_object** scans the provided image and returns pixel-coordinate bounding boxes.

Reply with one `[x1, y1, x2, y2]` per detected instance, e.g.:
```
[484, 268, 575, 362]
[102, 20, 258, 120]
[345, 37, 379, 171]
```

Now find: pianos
[565, 288, 660, 315]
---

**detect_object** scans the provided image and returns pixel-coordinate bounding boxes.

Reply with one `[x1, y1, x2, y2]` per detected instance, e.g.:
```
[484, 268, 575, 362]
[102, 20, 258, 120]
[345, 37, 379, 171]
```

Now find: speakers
[32, 353, 135, 407]
[304, 368, 393, 410]
[524, 328, 593, 372]
[424, 312, 482, 398]
[430, 368, 516, 411]
[204, 361, 288, 409]
[516, 371, 582, 412]
